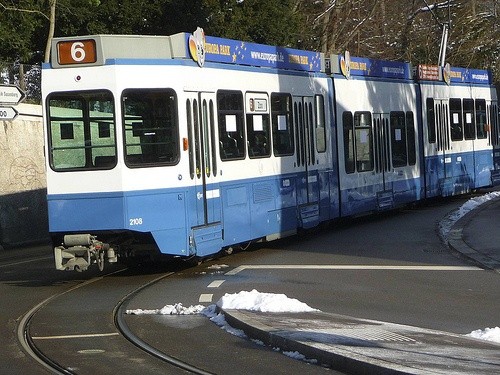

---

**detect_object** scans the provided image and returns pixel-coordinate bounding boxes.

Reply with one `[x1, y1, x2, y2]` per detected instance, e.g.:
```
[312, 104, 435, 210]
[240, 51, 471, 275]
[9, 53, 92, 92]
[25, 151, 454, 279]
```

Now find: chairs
[218, 129, 291, 159]
[451, 122, 486, 140]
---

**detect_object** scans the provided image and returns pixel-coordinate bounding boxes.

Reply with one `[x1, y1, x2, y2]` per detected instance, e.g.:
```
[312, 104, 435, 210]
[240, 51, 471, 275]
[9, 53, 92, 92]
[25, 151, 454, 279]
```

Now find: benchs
[95, 153, 155, 166]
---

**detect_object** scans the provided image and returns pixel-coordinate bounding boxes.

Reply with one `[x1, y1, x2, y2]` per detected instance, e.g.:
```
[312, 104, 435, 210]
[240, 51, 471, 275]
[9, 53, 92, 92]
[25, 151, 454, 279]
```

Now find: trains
[41, 32, 500, 273]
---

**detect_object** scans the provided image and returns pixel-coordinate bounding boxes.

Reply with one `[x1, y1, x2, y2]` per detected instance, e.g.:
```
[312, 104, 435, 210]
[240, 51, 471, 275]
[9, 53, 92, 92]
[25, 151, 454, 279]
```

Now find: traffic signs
[0, 85, 27, 105]
[0, 106, 19, 121]
